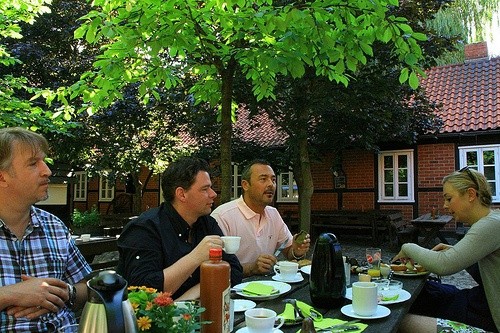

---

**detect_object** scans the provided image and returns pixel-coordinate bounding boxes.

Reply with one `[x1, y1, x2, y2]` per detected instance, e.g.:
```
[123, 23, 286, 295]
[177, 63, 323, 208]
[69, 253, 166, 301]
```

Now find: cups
[273, 261, 299, 279]
[352, 281, 379, 316]
[76, 234, 90, 245]
[366, 247, 381, 280]
[219, 236, 242, 255]
[244, 308, 284, 333]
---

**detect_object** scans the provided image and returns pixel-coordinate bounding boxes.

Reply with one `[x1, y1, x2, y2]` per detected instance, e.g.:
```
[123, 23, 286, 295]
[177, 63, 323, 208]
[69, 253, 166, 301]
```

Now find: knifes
[274, 238, 290, 257]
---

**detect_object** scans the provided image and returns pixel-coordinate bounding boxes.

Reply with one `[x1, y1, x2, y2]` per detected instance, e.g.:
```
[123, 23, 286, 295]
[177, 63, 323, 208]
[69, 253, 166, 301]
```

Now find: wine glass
[380, 256, 392, 279]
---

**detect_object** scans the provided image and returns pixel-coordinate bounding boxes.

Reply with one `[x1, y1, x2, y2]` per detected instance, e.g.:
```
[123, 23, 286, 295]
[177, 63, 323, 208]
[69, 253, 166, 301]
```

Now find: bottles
[199, 248, 231, 333]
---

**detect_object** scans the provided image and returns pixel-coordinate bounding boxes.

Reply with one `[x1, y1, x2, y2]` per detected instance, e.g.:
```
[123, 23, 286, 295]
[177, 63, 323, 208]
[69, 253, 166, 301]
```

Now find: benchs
[456, 226, 470, 242]
[282, 209, 401, 244]
[389, 213, 418, 250]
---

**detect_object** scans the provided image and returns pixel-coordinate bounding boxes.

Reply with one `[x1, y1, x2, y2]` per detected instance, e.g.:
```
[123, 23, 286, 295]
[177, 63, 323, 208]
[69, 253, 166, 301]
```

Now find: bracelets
[292, 248, 306, 259]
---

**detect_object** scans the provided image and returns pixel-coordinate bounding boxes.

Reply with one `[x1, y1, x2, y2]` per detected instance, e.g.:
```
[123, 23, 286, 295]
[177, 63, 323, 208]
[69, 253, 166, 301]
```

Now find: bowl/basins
[376, 280, 403, 301]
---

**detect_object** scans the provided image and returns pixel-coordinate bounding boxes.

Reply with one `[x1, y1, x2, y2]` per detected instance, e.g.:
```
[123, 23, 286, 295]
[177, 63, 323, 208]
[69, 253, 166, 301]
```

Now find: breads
[390, 265, 407, 271]
[414, 267, 426, 272]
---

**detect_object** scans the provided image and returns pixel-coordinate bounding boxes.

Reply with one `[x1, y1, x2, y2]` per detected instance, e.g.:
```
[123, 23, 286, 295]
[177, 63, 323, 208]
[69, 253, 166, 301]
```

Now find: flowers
[127, 286, 215, 333]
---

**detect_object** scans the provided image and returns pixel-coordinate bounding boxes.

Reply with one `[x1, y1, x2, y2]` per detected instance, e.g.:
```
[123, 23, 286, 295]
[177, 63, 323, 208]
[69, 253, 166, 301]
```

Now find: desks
[70, 234, 120, 269]
[231, 265, 431, 333]
[411, 212, 453, 247]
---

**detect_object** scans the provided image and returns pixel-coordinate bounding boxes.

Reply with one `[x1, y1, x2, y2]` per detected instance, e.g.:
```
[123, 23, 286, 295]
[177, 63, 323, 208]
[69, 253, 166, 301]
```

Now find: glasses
[458, 165, 479, 198]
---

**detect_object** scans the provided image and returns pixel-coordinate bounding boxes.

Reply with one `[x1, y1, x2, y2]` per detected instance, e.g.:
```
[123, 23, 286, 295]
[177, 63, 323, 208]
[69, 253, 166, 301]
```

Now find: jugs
[310, 232, 346, 308]
[77, 269, 141, 333]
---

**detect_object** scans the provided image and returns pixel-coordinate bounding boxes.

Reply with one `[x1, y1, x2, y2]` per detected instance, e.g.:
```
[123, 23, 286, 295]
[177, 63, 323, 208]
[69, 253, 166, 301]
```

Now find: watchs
[66, 283, 76, 305]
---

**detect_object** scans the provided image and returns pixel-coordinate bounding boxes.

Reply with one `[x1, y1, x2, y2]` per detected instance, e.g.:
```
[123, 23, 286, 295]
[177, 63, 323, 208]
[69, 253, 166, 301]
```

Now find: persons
[210, 159, 310, 275]
[117, 156, 243, 301]
[0, 126, 92, 333]
[391, 166, 500, 333]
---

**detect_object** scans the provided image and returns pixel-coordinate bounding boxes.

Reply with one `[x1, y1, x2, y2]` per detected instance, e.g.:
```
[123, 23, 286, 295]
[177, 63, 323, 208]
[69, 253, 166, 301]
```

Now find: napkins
[296, 318, 368, 333]
[277, 300, 323, 325]
[242, 282, 274, 297]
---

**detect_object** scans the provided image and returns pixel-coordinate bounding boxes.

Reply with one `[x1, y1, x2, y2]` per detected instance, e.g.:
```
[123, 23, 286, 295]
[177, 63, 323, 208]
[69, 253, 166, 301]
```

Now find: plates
[341, 304, 391, 319]
[300, 265, 311, 275]
[233, 299, 256, 313]
[393, 270, 430, 276]
[346, 287, 411, 304]
[272, 276, 304, 282]
[72, 236, 79, 239]
[233, 281, 291, 301]
[236, 327, 284, 333]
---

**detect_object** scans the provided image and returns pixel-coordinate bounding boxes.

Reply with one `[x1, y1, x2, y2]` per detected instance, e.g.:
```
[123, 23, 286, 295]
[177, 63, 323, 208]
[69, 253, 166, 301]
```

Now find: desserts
[294, 230, 308, 244]
